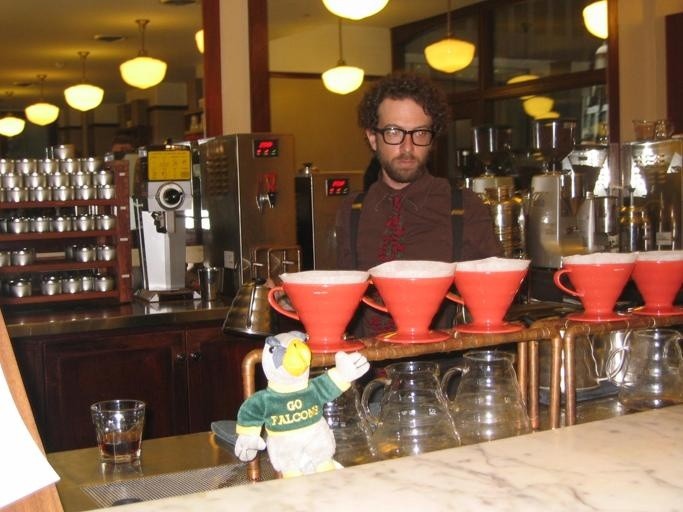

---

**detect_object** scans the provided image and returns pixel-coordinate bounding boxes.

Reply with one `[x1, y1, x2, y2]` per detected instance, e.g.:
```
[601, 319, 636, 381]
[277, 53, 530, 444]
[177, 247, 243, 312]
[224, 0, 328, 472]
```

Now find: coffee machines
[197, 133, 300, 294]
[135, 141, 202, 302]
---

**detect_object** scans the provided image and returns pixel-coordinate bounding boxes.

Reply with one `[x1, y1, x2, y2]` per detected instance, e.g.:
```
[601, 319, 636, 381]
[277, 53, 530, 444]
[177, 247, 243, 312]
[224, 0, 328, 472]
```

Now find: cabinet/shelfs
[2, 158, 131, 303]
[5, 302, 267, 453]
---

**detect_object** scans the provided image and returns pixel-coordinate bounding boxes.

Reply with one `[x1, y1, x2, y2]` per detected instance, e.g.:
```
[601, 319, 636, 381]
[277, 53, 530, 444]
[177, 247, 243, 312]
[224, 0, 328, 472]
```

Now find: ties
[361, 193, 406, 376]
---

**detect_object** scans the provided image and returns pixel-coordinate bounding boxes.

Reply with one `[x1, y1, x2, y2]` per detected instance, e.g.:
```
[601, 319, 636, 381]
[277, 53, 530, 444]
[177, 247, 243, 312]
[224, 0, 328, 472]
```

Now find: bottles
[655, 208, 676, 251]
[595, 196, 617, 233]
[620, 206, 636, 252]
[637, 210, 654, 251]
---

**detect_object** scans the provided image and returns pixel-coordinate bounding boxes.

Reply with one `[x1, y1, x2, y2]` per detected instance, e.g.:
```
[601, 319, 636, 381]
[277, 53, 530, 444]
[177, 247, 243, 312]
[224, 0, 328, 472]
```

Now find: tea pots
[219, 277, 274, 339]
[520, 314, 625, 391]
[606, 328, 683, 411]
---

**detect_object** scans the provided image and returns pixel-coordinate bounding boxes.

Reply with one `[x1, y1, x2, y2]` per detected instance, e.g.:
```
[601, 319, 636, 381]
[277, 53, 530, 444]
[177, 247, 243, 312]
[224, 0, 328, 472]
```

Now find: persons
[328, 68, 504, 341]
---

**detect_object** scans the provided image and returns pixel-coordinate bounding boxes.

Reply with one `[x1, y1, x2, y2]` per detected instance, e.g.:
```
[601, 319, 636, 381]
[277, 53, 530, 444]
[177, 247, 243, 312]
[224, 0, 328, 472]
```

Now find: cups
[630, 251, 682, 317]
[454, 257, 530, 334]
[307, 369, 380, 465]
[197, 267, 225, 301]
[361, 260, 455, 343]
[90, 399, 145, 462]
[267, 271, 369, 354]
[440, 351, 531, 447]
[655, 120, 675, 139]
[553, 253, 635, 321]
[633, 120, 655, 141]
[360, 361, 461, 460]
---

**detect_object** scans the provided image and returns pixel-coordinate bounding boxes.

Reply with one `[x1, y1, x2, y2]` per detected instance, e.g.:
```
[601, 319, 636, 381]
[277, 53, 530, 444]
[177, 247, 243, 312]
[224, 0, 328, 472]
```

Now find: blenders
[464, 124, 526, 248]
[531, 118, 587, 270]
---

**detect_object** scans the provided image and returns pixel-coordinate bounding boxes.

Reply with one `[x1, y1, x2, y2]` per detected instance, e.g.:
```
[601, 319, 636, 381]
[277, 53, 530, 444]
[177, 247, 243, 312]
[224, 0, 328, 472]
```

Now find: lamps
[26, 76, 59, 127]
[507, 22, 540, 100]
[423, 1, 476, 74]
[321, 18, 364, 95]
[119, 19, 168, 90]
[0, 91, 25, 138]
[63, 52, 104, 111]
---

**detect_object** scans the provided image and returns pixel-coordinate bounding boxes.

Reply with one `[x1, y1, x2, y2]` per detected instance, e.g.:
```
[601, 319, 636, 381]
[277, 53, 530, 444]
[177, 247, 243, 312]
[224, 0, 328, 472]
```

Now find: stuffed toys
[234, 330, 372, 479]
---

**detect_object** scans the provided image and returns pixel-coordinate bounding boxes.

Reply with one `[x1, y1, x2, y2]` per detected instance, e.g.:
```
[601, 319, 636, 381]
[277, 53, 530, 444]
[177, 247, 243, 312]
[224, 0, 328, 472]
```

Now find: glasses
[369, 128, 438, 146]
